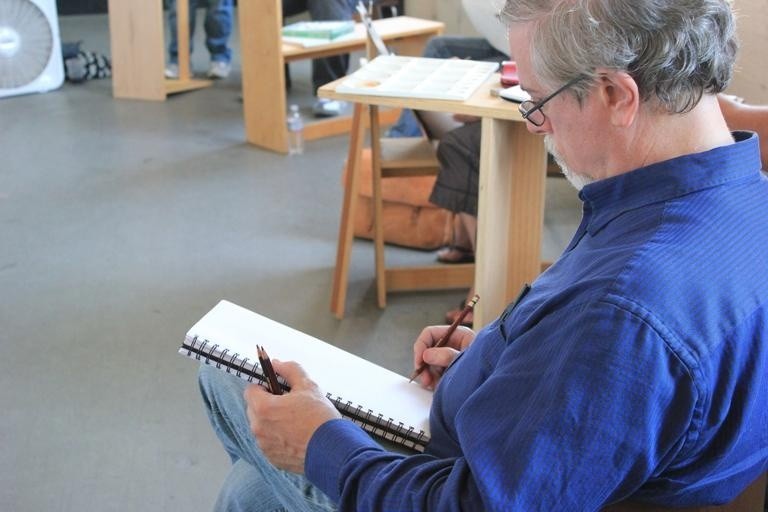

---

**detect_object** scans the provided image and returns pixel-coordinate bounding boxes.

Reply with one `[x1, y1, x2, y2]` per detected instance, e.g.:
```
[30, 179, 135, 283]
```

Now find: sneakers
[310, 99, 340, 115]
[167, 62, 192, 80]
[210, 60, 227, 78]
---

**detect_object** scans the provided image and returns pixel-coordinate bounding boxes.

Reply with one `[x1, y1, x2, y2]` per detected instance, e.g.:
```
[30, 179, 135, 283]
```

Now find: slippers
[435, 244, 477, 263]
[446, 299, 475, 327]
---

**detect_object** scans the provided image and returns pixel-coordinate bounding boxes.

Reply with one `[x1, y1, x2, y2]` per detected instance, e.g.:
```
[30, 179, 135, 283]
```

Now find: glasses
[520, 75, 581, 127]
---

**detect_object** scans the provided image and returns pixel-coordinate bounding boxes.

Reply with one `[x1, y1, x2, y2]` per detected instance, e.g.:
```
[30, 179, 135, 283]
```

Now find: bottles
[286, 103, 305, 158]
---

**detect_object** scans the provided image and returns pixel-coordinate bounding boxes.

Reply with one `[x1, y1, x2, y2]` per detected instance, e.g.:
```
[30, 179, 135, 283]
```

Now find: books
[177, 300, 436, 454]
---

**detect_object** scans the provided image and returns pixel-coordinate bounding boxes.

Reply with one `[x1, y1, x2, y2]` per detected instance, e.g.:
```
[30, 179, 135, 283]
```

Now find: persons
[386, 0, 517, 137]
[284, 0, 384, 119]
[427, 111, 482, 328]
[163, 0, 237, 80]
[193, 0, 768, 512]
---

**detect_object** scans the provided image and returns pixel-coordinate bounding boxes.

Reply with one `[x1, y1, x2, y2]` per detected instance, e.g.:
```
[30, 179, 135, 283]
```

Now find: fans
[0, 0, 66, 98]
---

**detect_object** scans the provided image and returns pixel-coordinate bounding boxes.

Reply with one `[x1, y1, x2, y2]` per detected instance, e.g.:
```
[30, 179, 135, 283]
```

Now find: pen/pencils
[408, 294, 479, 384]
[256, 345, 282, 395]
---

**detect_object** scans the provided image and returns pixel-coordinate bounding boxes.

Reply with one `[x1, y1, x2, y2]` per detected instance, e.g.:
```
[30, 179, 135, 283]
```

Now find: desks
[315, 51, 576, 320]
